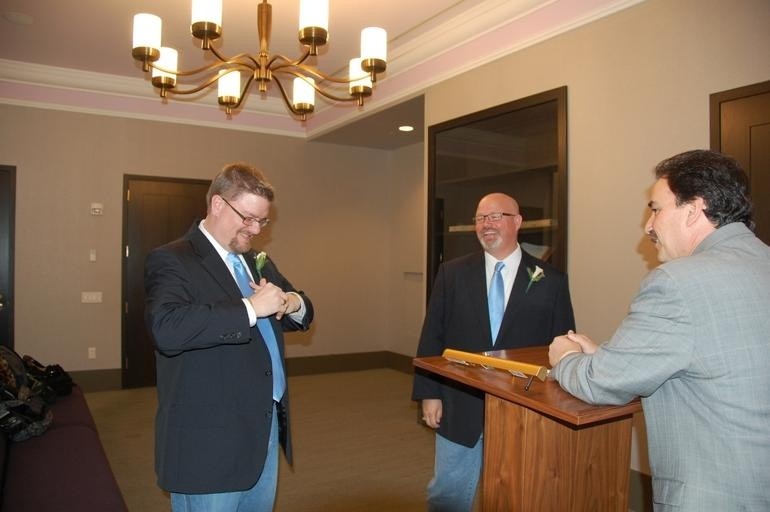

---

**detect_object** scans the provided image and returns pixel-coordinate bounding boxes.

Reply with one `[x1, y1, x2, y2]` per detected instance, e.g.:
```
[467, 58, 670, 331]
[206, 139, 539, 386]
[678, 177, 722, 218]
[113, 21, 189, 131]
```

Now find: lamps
[130, 1, 387, 120]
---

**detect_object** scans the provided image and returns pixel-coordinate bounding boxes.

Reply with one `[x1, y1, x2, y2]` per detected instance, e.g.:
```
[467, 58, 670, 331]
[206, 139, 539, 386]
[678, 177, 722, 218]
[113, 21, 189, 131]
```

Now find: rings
[422, 416, 427, 420]
[282, 298, 286, 305]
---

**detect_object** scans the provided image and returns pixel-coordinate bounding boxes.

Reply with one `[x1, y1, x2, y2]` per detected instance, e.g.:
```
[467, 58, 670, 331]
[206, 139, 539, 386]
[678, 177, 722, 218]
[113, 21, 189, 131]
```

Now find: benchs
[0, 343, 131, 511]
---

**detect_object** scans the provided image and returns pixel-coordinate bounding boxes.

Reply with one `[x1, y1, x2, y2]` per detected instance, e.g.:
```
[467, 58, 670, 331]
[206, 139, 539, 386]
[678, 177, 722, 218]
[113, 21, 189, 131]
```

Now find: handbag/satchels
[22, 353, 75, 397]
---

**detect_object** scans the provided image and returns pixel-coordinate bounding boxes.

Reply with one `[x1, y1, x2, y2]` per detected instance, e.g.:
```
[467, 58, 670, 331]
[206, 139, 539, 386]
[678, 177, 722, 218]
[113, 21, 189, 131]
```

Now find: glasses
[472, 212, 516, 226]
[221, 197, 270, 229]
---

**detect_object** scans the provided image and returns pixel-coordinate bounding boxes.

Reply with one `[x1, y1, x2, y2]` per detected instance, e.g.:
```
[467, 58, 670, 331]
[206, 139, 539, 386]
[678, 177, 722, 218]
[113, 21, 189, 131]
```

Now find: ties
[225, 252, 287, 403]
[487, 262, 505, 347]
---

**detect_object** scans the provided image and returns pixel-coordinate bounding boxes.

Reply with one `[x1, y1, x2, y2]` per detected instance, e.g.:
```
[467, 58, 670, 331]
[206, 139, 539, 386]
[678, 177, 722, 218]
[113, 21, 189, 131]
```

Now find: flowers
[252, 250, 269, 279]
[524, 263, 547, 294]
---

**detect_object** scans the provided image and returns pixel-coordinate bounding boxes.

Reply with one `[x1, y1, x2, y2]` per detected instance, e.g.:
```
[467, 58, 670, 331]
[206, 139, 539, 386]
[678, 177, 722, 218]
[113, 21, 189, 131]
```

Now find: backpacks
[0, 346, 55, 443]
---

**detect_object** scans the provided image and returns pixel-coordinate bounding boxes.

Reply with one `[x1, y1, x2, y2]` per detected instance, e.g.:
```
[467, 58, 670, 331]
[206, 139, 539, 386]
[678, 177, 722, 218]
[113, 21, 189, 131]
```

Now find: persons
[139, 164, 314, 510]
[410, 189, 577, 512]
[547, 146, 769, 512]
